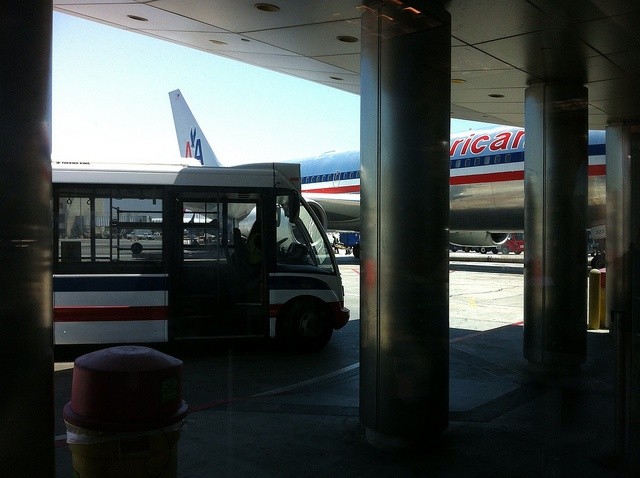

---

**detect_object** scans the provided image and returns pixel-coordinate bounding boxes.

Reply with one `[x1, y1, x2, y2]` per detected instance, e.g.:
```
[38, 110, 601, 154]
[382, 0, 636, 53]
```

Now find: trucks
[339, 232, 360, 259]
[449, 233, 524, 255]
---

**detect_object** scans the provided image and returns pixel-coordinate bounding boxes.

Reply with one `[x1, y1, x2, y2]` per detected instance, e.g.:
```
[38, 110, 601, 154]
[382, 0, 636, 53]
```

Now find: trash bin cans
[65, 348, 191, 476]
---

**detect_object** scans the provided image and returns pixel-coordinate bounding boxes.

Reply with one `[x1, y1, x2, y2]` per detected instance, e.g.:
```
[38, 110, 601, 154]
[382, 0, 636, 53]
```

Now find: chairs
[232, 226, 249, 261]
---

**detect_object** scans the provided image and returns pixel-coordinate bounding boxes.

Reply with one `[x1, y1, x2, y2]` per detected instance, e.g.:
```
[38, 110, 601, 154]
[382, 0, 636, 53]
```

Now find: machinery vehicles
[126, 230, 163, 239]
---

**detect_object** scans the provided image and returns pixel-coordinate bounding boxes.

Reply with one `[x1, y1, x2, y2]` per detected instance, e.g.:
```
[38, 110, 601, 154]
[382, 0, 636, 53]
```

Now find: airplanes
[167, 87, 607, 267]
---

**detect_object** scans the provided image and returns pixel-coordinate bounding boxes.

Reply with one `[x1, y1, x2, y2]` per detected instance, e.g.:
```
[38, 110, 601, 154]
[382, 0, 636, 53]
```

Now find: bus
[52, 162, 351, 355]
